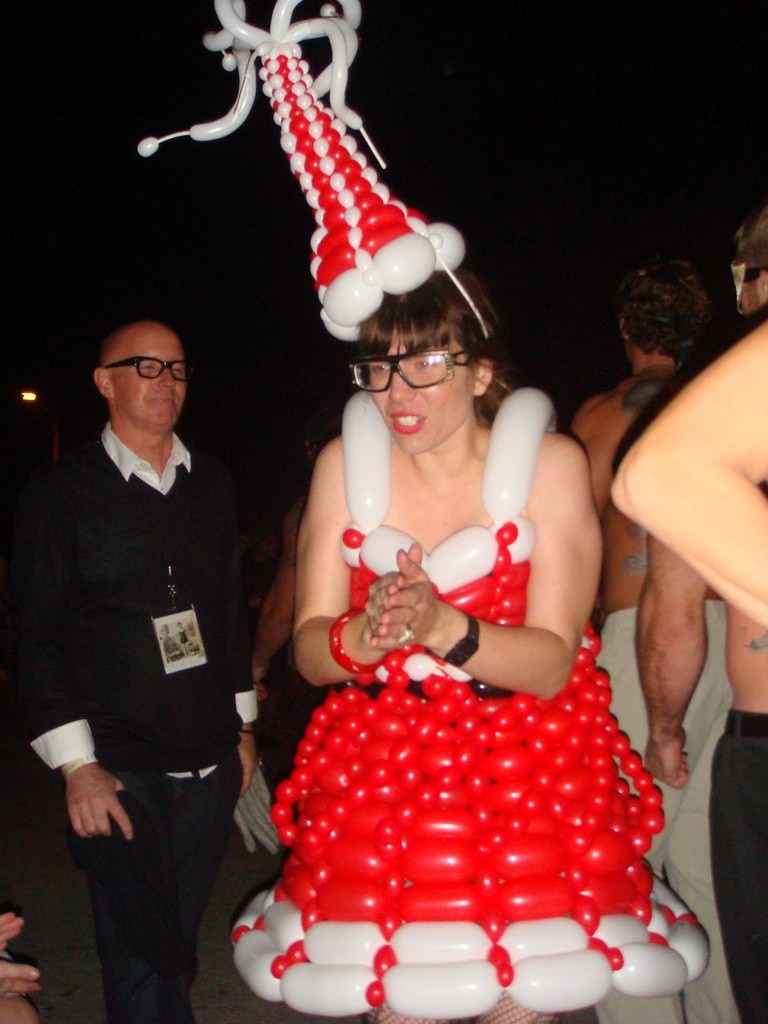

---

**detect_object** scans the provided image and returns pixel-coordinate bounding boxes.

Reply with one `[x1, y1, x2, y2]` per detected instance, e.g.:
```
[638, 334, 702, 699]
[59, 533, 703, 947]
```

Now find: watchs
[444, 613, 480, 666]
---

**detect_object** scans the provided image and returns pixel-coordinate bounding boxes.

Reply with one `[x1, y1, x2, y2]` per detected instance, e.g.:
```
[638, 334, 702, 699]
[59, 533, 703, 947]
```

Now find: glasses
[348, 349, 473, 392]
[105, 356, 194, 382]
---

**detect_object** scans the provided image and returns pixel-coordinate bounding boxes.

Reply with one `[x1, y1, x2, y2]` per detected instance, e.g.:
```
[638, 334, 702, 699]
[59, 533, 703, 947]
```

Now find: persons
[0, 913, 41, 1024]
[232, 263, 711, 1024]
[563, 255, 743, 1024]
[177, 622, 190, 657]
[634, 203, 768, 1024]
[8, 316, 258, 1024]
[608, 316, 768, 642]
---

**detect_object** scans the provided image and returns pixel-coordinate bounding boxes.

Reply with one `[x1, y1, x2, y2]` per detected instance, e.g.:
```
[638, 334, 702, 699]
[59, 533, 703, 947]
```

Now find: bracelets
[60, 754, 98, 776]
[240, 728, 254, 734]
[327, 607, 387, 674]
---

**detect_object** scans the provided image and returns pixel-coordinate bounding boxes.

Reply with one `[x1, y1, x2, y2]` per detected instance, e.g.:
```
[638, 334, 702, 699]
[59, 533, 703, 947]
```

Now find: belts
[723, 709, 768, 739]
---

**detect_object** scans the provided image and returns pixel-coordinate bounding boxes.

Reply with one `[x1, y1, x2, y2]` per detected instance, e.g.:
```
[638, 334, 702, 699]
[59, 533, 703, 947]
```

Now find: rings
[399, 625, 415, 643]
